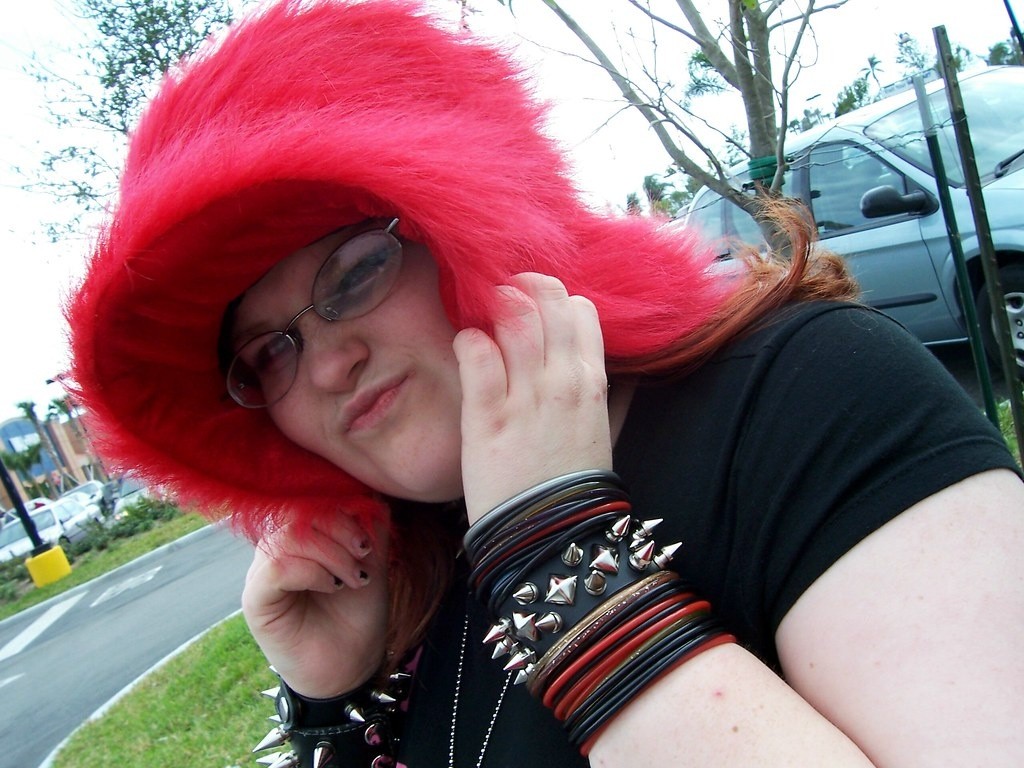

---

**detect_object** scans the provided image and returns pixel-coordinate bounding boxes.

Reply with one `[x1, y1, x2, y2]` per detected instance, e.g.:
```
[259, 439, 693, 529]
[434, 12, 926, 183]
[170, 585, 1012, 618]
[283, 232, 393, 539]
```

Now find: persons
[187, 98, 1023, 768]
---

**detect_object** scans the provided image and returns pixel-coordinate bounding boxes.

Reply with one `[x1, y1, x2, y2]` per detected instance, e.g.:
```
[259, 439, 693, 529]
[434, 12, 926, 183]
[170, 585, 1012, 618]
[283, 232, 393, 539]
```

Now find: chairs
[844, 159, 886, 222]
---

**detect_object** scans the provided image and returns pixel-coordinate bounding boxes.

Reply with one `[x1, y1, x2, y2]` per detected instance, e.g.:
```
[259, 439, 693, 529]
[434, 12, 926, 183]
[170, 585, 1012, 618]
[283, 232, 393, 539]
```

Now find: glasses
[219, 216, 408, 410]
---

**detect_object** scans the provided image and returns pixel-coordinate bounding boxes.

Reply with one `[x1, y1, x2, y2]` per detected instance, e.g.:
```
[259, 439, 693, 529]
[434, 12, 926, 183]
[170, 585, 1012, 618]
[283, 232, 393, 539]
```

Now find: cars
[657, 63, 1023, 381]
[0, 465, 184, 568]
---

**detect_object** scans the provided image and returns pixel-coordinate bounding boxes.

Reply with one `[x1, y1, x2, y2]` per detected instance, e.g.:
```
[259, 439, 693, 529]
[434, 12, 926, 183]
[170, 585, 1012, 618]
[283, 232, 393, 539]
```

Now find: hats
[56, 0, 737, 562]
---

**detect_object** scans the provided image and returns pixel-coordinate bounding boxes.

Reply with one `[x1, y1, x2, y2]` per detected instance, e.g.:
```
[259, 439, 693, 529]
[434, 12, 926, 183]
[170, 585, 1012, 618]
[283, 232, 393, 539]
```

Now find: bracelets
[249, 645, 426, 768]
[454, 465, 738, 758]
